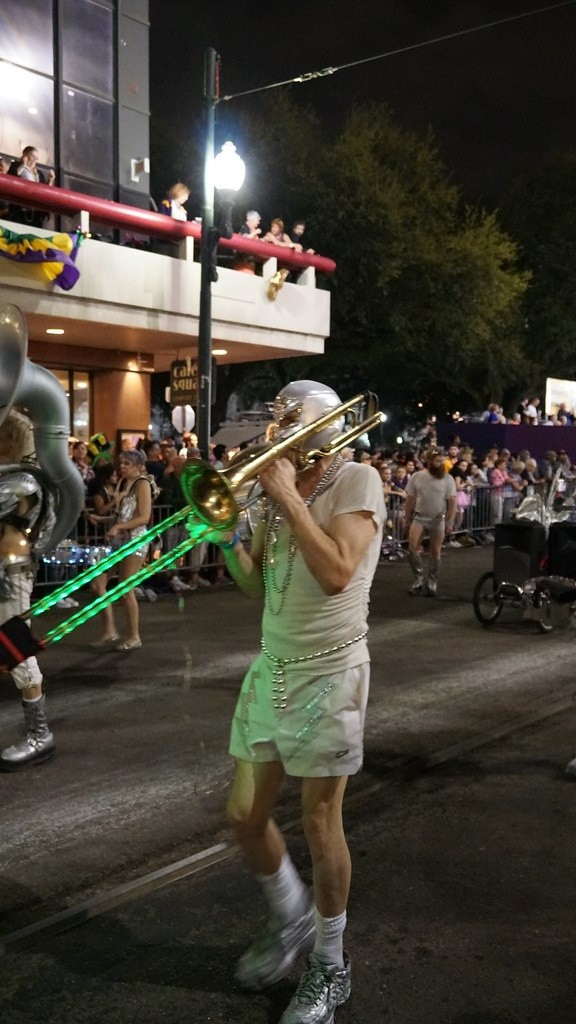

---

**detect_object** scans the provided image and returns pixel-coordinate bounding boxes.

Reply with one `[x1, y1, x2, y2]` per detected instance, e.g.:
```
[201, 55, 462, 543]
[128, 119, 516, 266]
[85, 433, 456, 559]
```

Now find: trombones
[0, 388, 392, 673]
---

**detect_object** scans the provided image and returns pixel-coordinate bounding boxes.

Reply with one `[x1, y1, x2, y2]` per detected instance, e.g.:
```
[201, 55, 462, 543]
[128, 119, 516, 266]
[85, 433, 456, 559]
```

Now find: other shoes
[55, 599, 71, 609]
[87, 633, 120, 648]
[63, 596, 79, 607]
[167, 575, 190, 592]
[447, 531, 494, 547]
[214, 576, 234, 586]
[113, 639, 143, 651]
[191, 576, 211, 587]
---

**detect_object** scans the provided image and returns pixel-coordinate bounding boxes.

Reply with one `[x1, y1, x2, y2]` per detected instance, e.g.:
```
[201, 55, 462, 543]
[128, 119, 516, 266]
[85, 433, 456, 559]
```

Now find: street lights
[199, 139, 246, 464]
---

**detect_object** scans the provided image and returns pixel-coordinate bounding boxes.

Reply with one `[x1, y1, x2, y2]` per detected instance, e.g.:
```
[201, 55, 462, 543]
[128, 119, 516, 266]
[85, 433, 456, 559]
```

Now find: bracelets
[223, 535, 239, 548]
[291, 243, 296, 249]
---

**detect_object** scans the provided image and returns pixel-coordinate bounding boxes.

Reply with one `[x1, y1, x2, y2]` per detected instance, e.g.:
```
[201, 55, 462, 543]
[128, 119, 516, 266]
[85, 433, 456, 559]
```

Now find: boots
[406, 555, 426, 595]
[425, 557, 442, 595]
[0, 692, 56, 769]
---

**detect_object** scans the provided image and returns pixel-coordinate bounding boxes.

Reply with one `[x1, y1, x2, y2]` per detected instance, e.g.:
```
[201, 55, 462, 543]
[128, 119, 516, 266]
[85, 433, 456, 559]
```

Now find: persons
[234, 211, 262, 274]
[285, 220, 314, 283]
[88, 447, 153, 650]
[33, 432, 255, 612]
[403, 447, 457, 595]
[184, 381, 387, 1024]
[479, 395, 576, 426]
[261, 216, 303, 279]
[149, 181, 190, 254]
[0, 408, 56, 767]
[0, 145, 55, 229]
[341, 413, 576, 554]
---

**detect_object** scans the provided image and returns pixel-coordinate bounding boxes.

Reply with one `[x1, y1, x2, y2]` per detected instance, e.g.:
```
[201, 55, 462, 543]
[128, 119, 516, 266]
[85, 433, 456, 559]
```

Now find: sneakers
[277, 946, 352, 1024]
[238, 893, 318, 990]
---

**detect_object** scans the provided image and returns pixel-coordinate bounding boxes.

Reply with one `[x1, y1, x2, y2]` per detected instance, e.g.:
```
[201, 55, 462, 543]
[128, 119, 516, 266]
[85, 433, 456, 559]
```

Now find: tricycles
[468, 519, 576, 635]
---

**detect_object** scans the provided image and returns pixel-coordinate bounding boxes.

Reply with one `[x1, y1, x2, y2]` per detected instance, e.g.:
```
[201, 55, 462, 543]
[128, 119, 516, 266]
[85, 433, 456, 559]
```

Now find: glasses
[431, 448, 441, 456]
[366, 456, 372, 461]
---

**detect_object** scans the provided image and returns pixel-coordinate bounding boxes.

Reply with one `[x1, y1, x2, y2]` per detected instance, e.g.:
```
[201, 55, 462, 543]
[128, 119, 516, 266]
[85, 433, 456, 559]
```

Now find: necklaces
[261, 455, 342, 615]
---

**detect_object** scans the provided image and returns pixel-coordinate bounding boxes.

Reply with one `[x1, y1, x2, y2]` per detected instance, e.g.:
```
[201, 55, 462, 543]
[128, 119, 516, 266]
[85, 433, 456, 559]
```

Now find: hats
[501, 449, 510, 454]
[340, 447, 356, 454]
